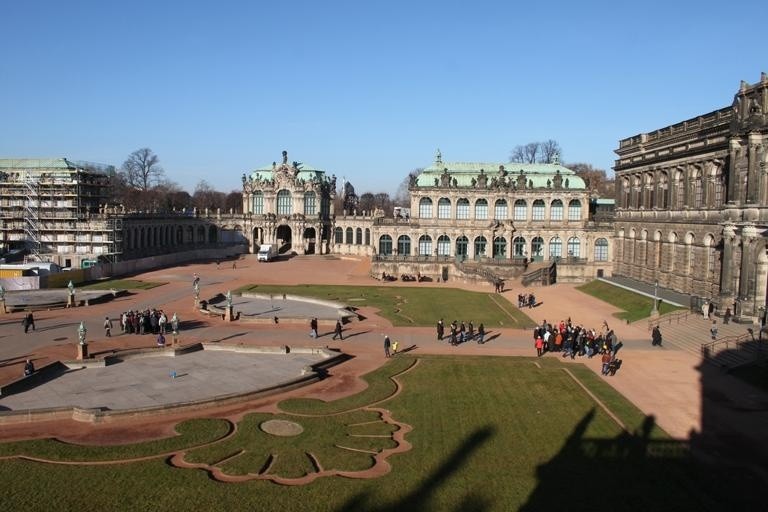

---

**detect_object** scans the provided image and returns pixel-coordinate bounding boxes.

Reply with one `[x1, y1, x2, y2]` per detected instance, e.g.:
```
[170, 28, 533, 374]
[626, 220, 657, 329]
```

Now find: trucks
[256, 244, 278, 262]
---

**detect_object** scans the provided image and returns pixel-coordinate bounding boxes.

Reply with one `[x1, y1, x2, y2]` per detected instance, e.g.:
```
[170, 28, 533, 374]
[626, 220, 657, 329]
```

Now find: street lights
[654, 280, 659, 310]
